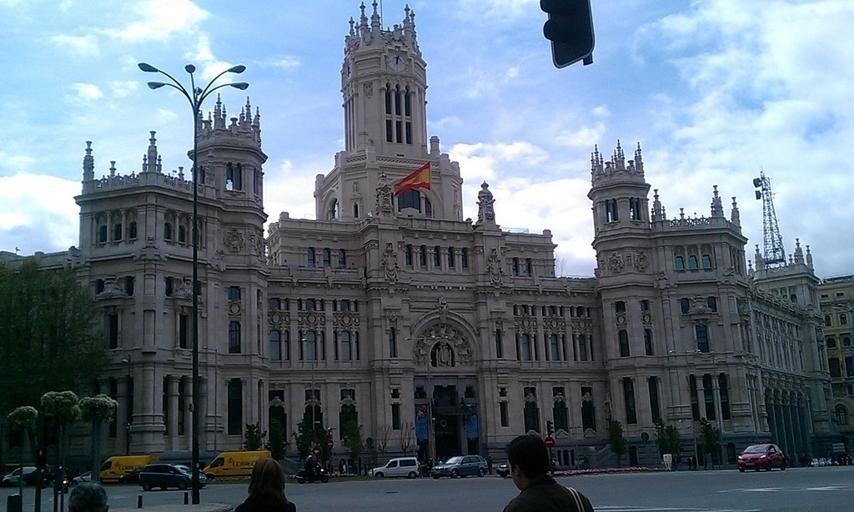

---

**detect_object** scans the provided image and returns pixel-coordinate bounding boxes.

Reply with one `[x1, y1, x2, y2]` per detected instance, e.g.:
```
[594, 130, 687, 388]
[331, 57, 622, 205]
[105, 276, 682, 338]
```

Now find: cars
[73, 471, 91, 483]
[368, 456, 419, 479]
[1, 466, 51, 487]
[737, 444, 785, 472]
[139, 463, 207, 490]
[431, 455, 488, 479]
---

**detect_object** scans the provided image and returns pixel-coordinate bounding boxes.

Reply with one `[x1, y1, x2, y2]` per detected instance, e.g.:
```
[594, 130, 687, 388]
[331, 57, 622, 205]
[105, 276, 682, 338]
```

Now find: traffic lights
[539, 0, 595, 69]
[547, 420, 554, 435]
[36, 443, 48, 463]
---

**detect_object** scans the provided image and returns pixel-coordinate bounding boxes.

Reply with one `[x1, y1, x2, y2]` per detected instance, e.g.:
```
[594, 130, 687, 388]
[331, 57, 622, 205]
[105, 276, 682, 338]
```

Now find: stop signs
[544, 437, 555, 447]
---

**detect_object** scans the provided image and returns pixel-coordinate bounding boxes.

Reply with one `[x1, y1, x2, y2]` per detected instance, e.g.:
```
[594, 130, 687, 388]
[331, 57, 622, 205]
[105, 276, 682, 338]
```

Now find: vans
[202, 451, 272, 481]
[99, 456, 159, 481]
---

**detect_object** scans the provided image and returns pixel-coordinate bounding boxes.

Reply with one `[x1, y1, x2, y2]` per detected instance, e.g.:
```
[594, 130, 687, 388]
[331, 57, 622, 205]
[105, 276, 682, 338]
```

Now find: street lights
[138, 62, 249, 504]
[202, 346, 218, 456]
[300, 337, 314, 431]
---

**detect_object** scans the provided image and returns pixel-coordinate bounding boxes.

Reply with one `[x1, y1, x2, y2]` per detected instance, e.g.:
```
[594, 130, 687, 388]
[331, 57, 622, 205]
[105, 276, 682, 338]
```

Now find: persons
[68, 484, 109, 512]
[419, 456, 438, 479]
[687, 457, 692, 471]
[234, 458, 297, 512]
[503, 435, 596, 511]
[304, 455, 315, 483]
[487, 455, 494, 474]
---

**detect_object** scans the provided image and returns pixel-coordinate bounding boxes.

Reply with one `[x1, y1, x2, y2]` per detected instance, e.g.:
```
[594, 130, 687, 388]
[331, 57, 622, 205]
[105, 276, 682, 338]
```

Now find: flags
[393, 160, 431, 197]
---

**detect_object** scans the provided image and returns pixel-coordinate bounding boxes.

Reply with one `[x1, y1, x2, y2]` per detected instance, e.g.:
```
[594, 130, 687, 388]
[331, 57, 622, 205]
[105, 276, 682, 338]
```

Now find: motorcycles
[53, 475, 68, 494]
[295, 464, 329, 484]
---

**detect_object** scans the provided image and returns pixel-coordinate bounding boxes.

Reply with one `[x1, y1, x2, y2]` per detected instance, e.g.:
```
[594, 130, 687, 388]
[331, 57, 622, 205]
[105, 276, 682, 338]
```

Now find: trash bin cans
[7, 495, 18, 512]
